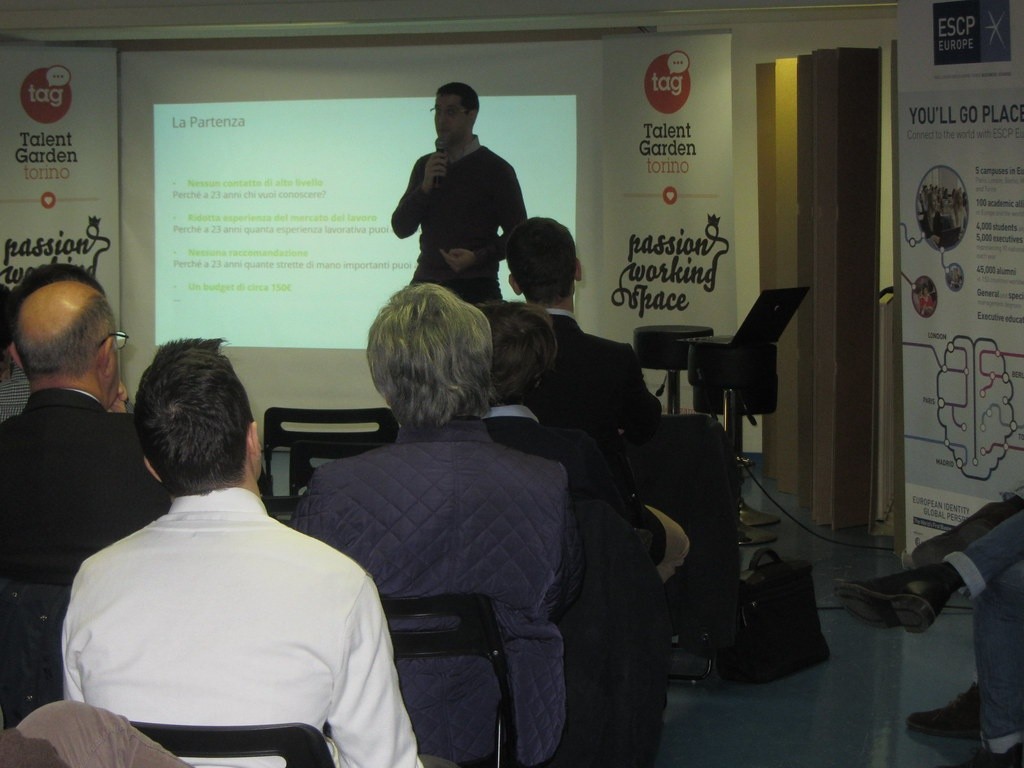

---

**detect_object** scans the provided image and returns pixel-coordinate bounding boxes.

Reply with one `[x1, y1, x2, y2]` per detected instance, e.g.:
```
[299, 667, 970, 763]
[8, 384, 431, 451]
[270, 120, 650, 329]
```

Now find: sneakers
[904, 684, 980, 739]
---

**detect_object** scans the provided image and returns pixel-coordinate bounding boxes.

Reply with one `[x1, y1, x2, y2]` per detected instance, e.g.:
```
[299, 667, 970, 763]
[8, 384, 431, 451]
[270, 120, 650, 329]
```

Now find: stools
[632, 321, 785, 542]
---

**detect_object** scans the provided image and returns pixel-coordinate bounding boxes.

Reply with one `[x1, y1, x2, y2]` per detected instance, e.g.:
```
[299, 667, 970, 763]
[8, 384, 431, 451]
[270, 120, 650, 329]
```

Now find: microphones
[433, 137, 448, 192]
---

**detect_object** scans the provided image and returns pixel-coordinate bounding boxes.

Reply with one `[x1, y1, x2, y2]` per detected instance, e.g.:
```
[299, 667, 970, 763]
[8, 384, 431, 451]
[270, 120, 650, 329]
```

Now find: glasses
[428, 106, 469, 117]
[99, 330, 129, 350]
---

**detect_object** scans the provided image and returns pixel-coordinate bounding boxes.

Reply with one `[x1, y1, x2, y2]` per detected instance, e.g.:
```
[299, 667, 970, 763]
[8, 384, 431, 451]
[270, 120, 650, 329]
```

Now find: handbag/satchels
[735, 548, 831, 682]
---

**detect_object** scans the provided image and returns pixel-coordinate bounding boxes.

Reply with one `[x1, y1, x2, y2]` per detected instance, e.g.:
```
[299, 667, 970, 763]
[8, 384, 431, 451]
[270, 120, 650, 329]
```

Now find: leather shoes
[833, 570, 949, 635]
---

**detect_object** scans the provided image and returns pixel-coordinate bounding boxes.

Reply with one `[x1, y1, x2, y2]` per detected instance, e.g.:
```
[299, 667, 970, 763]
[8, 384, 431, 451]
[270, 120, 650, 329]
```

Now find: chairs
[377, 592, 523, 768]
[257, 403, 402, 532]
[121, 722, 337, 768]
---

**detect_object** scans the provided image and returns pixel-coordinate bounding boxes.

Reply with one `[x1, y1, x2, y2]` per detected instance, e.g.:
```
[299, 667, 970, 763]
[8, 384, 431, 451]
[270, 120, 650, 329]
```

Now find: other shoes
[944, 742, 1024, 766]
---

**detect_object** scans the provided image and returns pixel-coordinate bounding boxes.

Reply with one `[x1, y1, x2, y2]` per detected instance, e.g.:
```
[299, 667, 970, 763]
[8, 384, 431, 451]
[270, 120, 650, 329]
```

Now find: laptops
[676, 285, 811, 346]
[939, 227, 961, 249]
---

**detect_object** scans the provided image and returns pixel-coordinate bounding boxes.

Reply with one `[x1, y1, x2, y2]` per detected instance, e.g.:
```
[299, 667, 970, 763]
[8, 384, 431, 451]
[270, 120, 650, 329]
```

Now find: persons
[912, 182, 967, 316]
[471, 299, 692, 582]
[828, 504, 1024, 768]
[0, 280, 173, 587]
[0, 698, 198, 768]
[390, 81, 532, 299]
[285, 278, 585, 766]
[1, 262, 138, 422]
[60, 337, 458, 767]
[907, 485, 1024, 741]
[505, 215, 664, 495]
